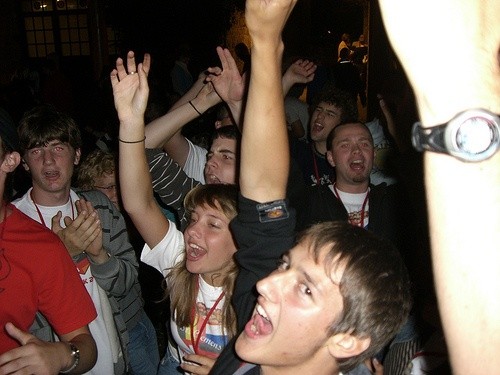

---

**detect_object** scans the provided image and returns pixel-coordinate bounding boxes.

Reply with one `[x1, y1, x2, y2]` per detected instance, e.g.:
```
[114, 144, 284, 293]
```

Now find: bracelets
[188, 100, 203, 116]
[117, 136, 146, 145]
[106, 253, 112, 258]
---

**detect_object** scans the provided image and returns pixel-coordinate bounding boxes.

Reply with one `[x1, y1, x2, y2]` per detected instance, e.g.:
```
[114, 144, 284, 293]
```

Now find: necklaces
[333, 183, 369, 228]
[30, 190, 74, 228]
[311, 141, 323, 186]
[189, 274, 226, 355]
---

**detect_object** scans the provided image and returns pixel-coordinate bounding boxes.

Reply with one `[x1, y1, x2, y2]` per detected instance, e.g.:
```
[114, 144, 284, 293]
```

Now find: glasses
[93, 184, 120, 192]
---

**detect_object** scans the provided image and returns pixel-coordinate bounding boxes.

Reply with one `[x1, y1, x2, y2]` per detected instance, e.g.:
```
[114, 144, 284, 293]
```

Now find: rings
[128, 71, 137, 74]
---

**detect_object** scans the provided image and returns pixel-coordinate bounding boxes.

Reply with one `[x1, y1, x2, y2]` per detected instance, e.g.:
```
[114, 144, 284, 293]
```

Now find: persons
[144, 47, 250, 226]
[380, 0, 500, 375]
[9, 110, 139, 375]
[163, 71, 236, 186]
[78, 152, 159, 375]
[282, 33, 443, 375]
[207, 0, 412, 375]
[284, 95, 308, 144]
[0, 108, 98, 375]
[110, 51, 238, 375]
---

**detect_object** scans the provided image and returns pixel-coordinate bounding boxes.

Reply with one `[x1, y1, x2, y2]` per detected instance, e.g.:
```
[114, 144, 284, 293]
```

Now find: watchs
[60, 343, 80, 374]
[412, 109, 500, 162]
